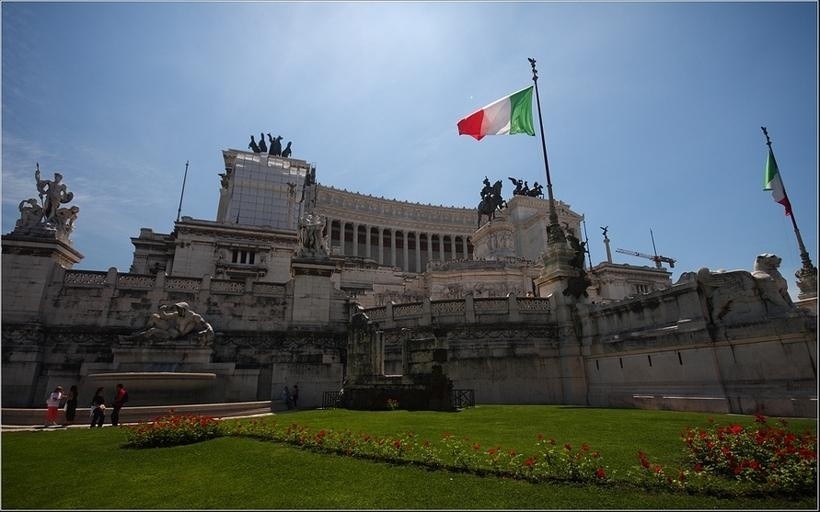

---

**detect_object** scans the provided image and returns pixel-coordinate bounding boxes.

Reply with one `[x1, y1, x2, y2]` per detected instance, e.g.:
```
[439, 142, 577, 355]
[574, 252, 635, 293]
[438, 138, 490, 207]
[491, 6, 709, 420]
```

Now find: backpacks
[120, 388, 130, 402]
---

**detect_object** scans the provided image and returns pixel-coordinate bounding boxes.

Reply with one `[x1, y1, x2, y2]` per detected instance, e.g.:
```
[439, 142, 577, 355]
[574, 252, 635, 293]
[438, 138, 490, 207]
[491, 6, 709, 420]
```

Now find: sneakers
[47, 421, 52, 425]
[52, 421, 57, 425]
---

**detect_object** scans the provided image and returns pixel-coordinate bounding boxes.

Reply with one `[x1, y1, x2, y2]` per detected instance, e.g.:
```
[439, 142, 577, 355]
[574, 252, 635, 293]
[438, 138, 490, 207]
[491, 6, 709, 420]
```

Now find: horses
[257, 132, 267, 152]
[536, 185, 544, 199]
[248, 135, 260, 153]
[477, 180, 508, 229]
[520, 181, 529, 195]
[528, 182, 538, 196]
[282, 142, 292, 158]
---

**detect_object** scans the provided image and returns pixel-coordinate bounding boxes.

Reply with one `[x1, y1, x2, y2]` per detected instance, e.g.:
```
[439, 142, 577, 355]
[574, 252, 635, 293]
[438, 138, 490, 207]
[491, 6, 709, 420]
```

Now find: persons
[111, 383, 128, 426]
[290, 384, 300, 409]
[116, 300, 213, 343]
[54, 203, 79, 235]
[62, 384, 81, 424]
[282, 385, 293, 409]
[35, 170, 69, 221]
[301, 214, 313, 248]
[308, 214, 327, 252]
[85, 386, 109, 430]
[44, 384, 65, 428]
[15, 197, 46, 228]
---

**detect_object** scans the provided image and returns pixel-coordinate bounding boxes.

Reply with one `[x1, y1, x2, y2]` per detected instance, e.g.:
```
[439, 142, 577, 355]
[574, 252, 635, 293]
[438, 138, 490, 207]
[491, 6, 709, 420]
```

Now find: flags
[763, 142, 794, 217]
[456, 84, 536, 142]
